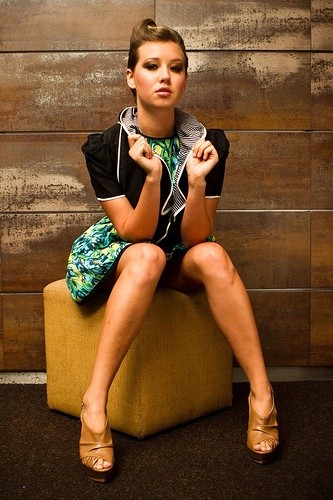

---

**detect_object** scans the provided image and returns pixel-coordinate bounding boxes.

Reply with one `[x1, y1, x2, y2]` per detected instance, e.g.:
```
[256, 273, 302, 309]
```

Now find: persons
[67, 18, 280, 484]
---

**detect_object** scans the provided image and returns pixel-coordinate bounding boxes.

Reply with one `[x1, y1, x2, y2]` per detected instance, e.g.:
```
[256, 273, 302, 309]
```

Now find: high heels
[78, 401, 115, 483]
[246, 391, 280, 464]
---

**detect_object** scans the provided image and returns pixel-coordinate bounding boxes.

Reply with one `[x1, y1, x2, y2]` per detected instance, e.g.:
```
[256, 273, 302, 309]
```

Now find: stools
[42, 278, 233, 437]
